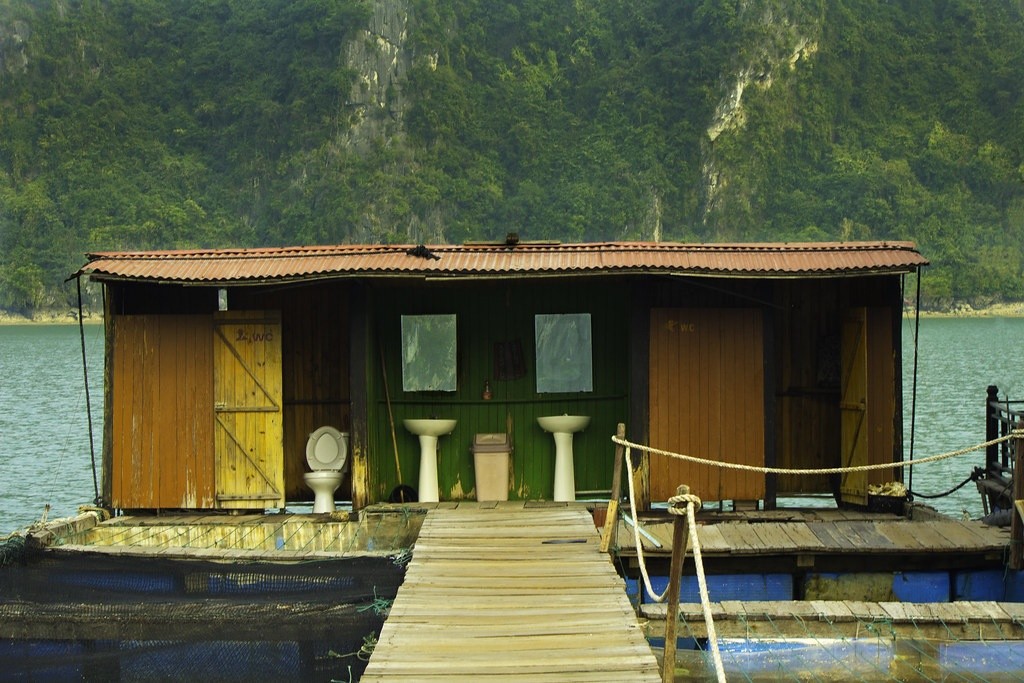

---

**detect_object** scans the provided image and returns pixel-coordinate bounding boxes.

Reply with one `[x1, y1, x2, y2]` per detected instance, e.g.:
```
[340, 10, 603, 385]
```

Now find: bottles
[483, 381, 492, 399]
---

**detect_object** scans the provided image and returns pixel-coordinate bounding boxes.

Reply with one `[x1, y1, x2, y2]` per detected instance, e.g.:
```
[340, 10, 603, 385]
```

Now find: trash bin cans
[472, 432, 512, 502]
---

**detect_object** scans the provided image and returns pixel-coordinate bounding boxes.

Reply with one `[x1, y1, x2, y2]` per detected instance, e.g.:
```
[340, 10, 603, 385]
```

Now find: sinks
[537, 414, 590, 432]
[403, 419, 457, 436]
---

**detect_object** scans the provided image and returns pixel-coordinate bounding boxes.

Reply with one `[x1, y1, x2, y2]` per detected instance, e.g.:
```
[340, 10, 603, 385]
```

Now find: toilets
[303, 426, 350, 514]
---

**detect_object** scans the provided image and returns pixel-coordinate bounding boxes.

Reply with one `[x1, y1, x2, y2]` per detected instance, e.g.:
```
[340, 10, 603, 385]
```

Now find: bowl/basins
[868, 494, 906, 514]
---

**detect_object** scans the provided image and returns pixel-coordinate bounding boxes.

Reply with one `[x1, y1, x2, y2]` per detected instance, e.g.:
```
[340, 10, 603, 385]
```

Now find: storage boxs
[867, 485, 907, 516]
